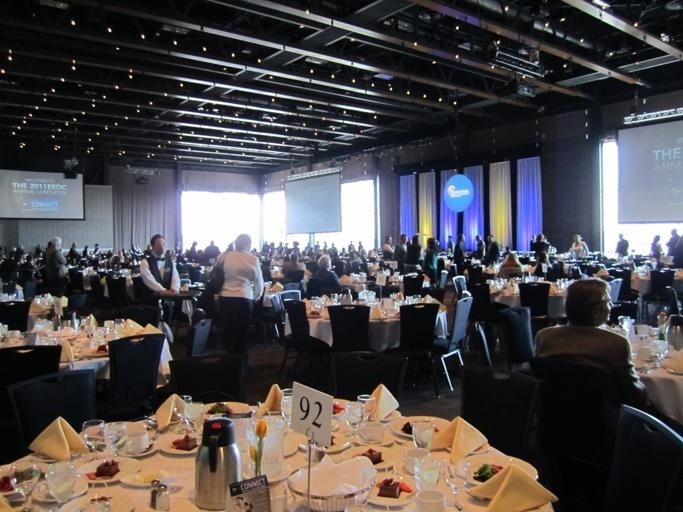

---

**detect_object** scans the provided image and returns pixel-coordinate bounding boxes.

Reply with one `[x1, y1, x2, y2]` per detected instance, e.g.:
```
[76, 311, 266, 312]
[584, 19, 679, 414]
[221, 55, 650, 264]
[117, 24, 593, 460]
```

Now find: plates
[241, 459, 298, 487]
[330, 401, 345, 418]
[300, 433, 351, 454]
[453, 453, 539, 485]
[203, 400, 250, 416]
[282, 437, 298, 457]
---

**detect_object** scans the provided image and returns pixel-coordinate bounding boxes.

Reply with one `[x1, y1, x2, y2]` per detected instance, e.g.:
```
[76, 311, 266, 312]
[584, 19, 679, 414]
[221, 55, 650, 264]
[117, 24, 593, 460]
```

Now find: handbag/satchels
[206, 266, 223, 295]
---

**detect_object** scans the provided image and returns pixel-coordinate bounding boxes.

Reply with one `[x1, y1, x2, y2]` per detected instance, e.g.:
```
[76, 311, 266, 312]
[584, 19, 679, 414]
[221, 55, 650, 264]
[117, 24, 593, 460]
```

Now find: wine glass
[0, 392, 201, 512]
[0, 315, 130, 365]
[345, 394, 463, 512]
[486, 274, 568, 294]
[306, 293, 424, 317]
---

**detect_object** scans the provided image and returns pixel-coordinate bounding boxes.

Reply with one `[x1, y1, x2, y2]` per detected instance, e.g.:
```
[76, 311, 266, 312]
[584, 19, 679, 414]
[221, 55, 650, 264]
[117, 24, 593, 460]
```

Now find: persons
[525, 280, 650, 502]
[1, 228, 680, 402]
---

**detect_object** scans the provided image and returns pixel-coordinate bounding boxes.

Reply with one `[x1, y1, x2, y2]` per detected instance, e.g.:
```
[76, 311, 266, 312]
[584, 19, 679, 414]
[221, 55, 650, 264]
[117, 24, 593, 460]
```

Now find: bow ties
[156, 258, 165, 261]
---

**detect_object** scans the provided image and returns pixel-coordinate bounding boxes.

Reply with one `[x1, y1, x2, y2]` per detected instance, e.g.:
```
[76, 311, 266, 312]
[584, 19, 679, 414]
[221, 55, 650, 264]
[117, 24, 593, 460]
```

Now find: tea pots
[192, 418, 242, 511]
[663, 314, 682, 351]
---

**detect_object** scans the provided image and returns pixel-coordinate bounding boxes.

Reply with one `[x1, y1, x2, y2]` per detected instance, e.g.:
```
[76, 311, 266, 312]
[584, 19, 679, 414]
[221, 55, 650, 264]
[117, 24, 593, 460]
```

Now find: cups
[615, 313, 674, 372]
[277, 387, 297, 425]
[245, 415, 284, 480]
